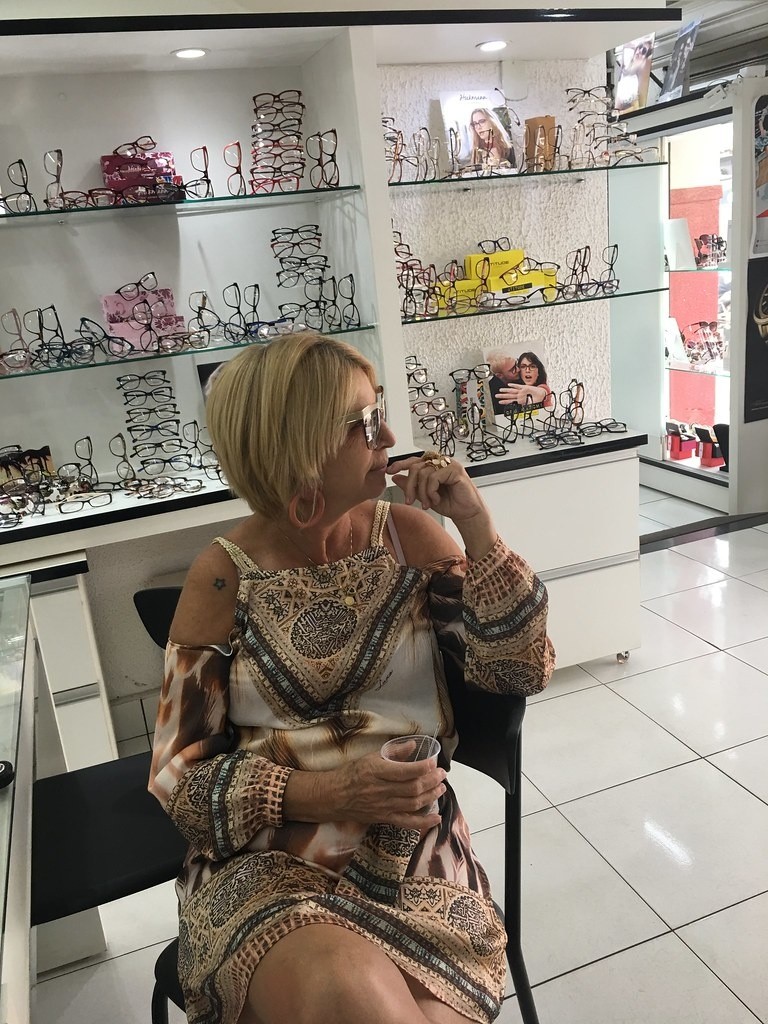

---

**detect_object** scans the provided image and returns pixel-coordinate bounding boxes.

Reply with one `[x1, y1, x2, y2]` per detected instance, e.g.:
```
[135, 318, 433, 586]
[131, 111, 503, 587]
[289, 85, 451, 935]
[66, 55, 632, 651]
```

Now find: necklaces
[261, 514, 356, 608]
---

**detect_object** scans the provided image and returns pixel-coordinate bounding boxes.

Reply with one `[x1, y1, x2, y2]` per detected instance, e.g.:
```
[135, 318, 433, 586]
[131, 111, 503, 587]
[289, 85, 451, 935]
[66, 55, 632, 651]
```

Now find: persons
[467, 105, 517, 169]
[486, 348, 530, 415]
[615, 41, 653, 114]
[495, 350, 554, 409]
[142, 330, 558, 1023]
[664, 35, 694, 95]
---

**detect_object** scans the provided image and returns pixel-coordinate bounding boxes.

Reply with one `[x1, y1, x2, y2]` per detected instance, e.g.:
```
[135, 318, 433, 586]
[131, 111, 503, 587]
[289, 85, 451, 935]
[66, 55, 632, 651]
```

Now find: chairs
[150, 642, 541, 1024]
[30, 586, 190, 928]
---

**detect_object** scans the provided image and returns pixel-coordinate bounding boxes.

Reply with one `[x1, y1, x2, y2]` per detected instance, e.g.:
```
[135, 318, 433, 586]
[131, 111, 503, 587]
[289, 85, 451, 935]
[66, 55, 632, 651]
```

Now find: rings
[419, 448, 452, 474]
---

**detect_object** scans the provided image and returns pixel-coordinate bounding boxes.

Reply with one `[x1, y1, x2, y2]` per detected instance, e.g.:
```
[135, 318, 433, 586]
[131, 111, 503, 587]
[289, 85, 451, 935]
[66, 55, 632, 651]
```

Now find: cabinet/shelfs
[608, 77, 767, 516]
[302, 8, 683, 477]
[388, 446, 642, 672]
[1, 10, 416, 567]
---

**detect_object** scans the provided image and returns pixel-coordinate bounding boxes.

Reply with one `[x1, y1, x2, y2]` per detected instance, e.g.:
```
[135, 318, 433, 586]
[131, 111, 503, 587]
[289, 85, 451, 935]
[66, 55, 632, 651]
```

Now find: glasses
[342, 385, 388, 450]
[638, 42, 647, 55]
[403, 345, 627, 465]
[0, 221, 374, 378]
[519, 364, 538, 371]
[0, 77, 349, 218]
[679, 313, 726, 368]
[691, 233, 729, 267]
[387, 214, 622, 323]
[509, 361, 518, 374]
[377, 83, 664, 186]
[0, 364, 236, 533]
[470, 119, 487, 129]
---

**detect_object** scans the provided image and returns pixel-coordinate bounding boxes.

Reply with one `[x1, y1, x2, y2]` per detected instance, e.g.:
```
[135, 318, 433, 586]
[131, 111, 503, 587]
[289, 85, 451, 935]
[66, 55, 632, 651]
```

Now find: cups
[380, 734, 439, 817]
